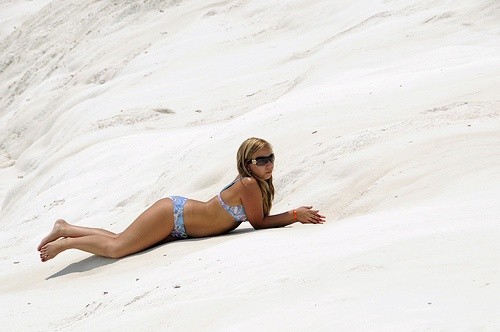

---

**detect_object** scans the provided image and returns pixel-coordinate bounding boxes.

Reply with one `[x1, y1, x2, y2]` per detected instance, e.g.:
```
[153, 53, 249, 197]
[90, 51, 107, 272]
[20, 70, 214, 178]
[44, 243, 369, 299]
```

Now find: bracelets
[292, 208, 297, 222]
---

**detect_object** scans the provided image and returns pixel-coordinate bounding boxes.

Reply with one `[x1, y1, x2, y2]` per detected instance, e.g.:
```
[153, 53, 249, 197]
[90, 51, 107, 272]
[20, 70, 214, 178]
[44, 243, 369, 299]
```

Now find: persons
[37, 136, 325, 262]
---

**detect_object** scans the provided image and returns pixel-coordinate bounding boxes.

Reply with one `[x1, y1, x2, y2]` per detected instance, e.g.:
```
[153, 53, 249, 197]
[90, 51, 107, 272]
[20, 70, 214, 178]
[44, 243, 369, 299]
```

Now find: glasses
[245, 154, 275, 166]
